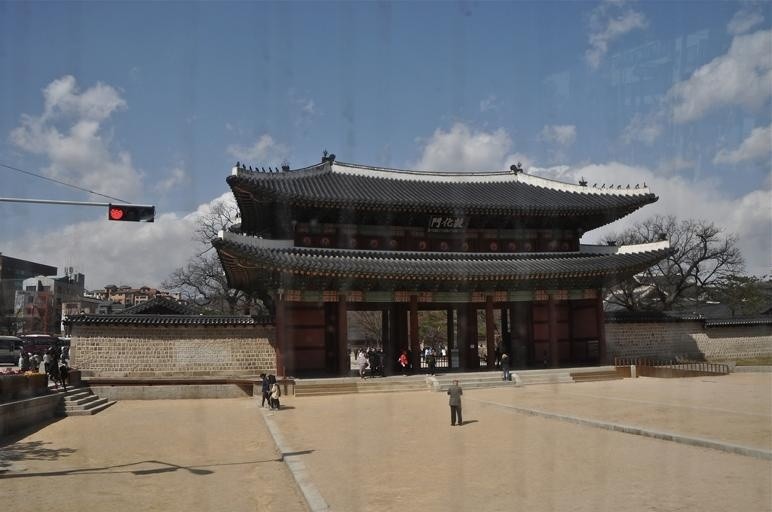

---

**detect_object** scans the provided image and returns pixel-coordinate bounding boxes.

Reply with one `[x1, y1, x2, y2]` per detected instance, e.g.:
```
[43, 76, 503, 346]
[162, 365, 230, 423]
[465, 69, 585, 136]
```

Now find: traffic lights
[108, 203, 156, 222]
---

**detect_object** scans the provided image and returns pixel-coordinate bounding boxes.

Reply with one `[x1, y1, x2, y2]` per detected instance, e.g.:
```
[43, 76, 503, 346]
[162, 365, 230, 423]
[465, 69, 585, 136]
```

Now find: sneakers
[458, 423, 463, 425]
[451, 424, 455, 426]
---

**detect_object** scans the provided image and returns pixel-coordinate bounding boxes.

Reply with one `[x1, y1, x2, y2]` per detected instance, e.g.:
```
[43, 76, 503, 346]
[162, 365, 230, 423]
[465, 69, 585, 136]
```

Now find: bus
[0, 334, 71, 367]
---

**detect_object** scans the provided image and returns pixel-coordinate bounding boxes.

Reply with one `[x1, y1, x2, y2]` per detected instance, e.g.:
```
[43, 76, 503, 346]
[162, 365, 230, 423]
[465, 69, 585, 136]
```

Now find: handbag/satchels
[365, 363, 369, 366]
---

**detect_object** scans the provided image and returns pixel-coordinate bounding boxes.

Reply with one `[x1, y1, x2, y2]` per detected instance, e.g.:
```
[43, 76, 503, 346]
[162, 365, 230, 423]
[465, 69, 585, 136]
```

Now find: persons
[347, 345, 453, 380]
[16, 342, 69, 392]
[260, 373, 281, 410]
[447, 380, 462, 426]
[502, 352, 510, 380]
